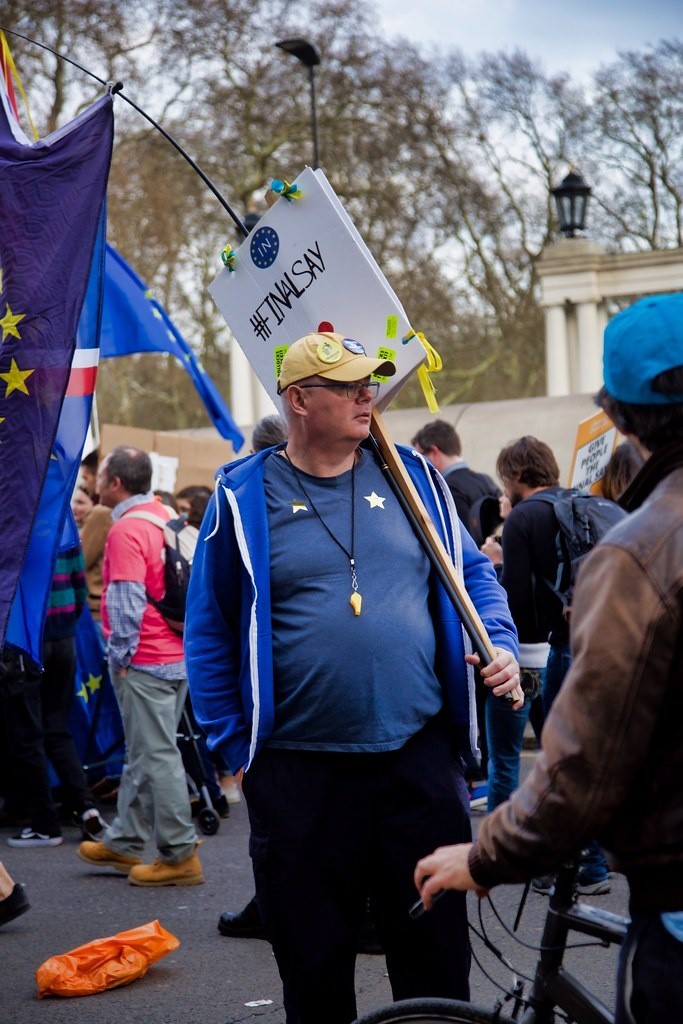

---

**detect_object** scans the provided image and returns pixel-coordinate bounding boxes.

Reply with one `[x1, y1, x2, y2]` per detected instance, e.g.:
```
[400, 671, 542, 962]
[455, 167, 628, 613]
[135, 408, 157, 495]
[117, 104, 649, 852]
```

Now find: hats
[277, 331, 396, 396]
[603, 292, 683, 405]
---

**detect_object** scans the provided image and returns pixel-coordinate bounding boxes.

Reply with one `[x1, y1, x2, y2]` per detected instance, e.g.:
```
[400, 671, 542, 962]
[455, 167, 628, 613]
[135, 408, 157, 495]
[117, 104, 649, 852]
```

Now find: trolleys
[73, 707, 220, 835]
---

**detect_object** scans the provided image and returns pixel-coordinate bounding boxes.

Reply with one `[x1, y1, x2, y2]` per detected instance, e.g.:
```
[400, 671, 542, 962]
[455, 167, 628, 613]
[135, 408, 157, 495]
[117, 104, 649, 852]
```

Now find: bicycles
[351, 838, 632, 1024]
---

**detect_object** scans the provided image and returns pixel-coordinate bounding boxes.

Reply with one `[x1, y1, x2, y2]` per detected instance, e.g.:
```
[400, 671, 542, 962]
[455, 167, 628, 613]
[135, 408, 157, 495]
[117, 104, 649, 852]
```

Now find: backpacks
[516, 488, 632, 614]
[116, 502, 200, 638]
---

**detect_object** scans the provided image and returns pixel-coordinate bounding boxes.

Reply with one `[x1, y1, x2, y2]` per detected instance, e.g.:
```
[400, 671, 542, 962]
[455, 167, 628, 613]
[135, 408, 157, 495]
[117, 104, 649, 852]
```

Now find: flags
[6, 199, 245, 662]
[0, 31, 115, 647]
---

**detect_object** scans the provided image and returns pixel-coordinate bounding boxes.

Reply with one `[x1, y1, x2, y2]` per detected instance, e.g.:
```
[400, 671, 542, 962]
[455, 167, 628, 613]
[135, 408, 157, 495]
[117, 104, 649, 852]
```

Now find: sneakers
[529, 854, 612, 895]
[465, 778, 489, 812]
[7, 825, 63, 848]
[75, 840, 141, 872]
[133, 841, 205, 887]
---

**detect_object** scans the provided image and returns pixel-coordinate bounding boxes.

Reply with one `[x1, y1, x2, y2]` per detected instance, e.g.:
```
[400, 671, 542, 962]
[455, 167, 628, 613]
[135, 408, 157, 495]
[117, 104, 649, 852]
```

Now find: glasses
[299, 381, 380, 400]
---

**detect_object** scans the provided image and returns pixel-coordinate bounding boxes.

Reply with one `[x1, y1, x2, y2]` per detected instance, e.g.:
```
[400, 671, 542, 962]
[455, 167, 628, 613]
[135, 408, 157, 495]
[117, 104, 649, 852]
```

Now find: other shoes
[220, 782, 240, 803]
[72, 802, 111, 841]
[191, 791, 229, 817]
[0, 883, 31, 927]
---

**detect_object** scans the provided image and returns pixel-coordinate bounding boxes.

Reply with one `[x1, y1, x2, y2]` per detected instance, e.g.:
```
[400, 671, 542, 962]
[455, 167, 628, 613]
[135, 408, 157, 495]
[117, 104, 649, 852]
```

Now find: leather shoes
[217, 898, 270, 938]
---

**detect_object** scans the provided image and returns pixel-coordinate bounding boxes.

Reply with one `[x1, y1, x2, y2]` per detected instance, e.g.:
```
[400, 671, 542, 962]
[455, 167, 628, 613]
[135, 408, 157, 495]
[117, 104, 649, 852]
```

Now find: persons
[603, 438, 645, 504]
[69, 449, 242, 818]
[413, 292, 683, 1024]
[251, 414, 288, 452]
[493, 486, 512, 537]
[182, 332, 525, 1024]
[75, 445, 206, 887]
[0, 861, 32, 927]
[411, 419, 503, 808]
[5, 542, 111, 848]
[480, 436, 630, 895]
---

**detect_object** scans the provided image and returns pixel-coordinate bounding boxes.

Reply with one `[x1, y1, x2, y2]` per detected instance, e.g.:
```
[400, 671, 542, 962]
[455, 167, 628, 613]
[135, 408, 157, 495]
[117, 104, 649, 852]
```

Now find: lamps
[549, 168, 592, 238]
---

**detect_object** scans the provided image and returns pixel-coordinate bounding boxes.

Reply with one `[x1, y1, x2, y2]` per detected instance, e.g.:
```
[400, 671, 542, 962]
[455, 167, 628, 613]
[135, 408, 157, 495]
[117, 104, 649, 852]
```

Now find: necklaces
[283, 444, 362, 615]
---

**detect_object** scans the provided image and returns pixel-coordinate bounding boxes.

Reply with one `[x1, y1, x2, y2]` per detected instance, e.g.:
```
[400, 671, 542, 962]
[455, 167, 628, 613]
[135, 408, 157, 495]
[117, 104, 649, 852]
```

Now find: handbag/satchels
[35, 920, 181, 998]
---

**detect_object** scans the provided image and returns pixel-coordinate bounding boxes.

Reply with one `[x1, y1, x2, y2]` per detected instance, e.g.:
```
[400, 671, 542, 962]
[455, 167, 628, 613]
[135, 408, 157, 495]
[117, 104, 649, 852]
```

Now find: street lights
[274, 38, 321, 170]
